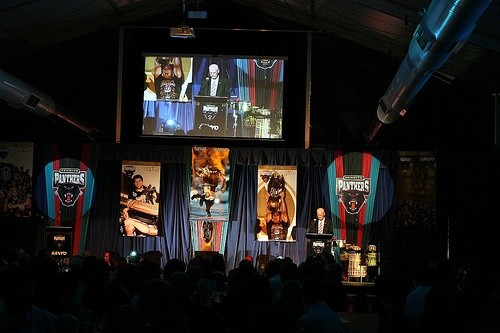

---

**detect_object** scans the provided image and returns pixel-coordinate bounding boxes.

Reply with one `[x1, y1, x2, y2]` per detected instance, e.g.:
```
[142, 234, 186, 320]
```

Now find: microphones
[313, 219, 317, 225]
[206, 77, 209, 85]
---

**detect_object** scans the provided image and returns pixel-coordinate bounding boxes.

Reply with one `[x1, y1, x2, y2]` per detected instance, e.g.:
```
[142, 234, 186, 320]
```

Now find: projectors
[170, 28, 195, 38]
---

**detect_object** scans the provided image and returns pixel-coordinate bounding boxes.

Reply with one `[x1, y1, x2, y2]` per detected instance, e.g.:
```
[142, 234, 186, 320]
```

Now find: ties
[211, 81, 216, 96]
[318, 222, 323, 234]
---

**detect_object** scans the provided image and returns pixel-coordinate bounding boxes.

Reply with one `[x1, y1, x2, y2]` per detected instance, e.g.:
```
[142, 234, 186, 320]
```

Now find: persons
[192, 166, 227, 218]
[0, 241, 500, 333]
[120, 174, 160, 236]
[264, 192, 290, 241]
[198, 64, 231, 100]
[153, 56, 185, 100]
[0, 142, 31, 216]
[308, 208, 333, 234]
